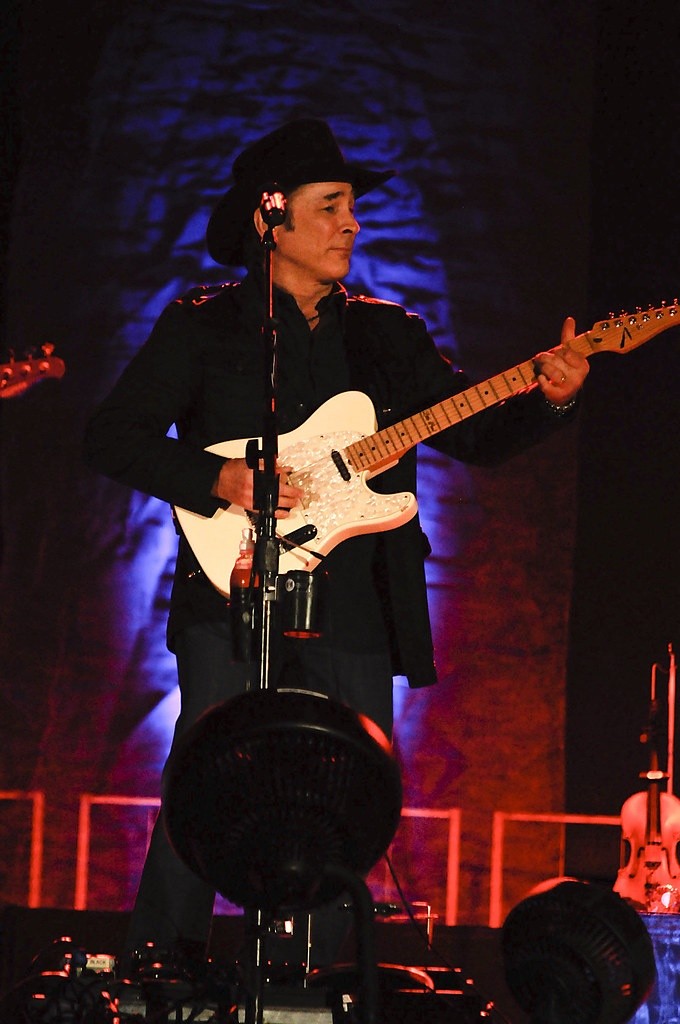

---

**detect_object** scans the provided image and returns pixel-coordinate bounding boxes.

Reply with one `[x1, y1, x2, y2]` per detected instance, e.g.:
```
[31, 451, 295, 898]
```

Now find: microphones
[254, 181, 288, 225]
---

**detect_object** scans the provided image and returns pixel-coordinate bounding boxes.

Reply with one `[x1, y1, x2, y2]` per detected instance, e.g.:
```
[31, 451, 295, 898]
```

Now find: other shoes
[133, 950, 195, 999]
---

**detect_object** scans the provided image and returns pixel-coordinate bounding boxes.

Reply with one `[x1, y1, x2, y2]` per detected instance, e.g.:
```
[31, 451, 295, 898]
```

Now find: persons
[82, 114, 592, 1009]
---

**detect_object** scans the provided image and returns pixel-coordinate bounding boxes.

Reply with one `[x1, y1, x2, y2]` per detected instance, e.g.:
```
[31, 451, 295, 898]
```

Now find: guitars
[0, 342, 66, 400]
[172, 298, 679, 605]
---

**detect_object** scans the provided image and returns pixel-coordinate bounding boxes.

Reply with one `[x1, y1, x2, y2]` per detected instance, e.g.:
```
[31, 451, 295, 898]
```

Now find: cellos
[611, 700, 680, 916]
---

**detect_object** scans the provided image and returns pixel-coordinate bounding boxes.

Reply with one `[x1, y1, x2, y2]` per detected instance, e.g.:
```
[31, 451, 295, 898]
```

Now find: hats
[206, 117, 399, 270]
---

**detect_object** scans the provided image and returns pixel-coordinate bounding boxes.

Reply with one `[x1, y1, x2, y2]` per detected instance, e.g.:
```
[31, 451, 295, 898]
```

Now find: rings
[558, 370, 567, 385]
